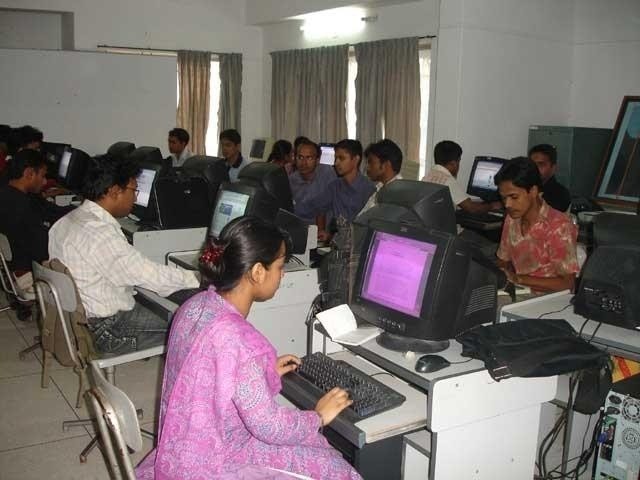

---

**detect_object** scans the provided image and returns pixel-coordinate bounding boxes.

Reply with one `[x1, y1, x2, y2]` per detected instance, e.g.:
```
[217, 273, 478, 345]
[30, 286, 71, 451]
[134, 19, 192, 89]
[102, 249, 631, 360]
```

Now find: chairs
[84, 360, 143, 479]
[0, 234, 35, 320]
[31, 259, 166, 408]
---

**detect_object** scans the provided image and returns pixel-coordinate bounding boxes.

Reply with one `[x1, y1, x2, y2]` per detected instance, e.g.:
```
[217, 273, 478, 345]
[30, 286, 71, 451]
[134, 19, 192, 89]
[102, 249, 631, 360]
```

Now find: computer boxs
[318, 425, 403, 479]
[591, 371, 640, 479]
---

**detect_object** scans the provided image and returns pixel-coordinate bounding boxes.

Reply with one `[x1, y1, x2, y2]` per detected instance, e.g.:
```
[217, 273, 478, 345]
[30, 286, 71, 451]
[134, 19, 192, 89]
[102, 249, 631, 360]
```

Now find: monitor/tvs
[348, 218, 498, 353]
[131, 161, 162, 221]
[574, 213, 640, 331]
[318, 143, 336, 167]
[466, 156, 508, 202]
[248, 137, 275, 162]
[108, 142, 136, 159]
[183, 155, 229, 185]
[130, 146, 162, 161]
[239, 162, 294, 213]
[352, 179, 458, 256]
[206, 183, 275, 244]
[55, 146, 92, 188]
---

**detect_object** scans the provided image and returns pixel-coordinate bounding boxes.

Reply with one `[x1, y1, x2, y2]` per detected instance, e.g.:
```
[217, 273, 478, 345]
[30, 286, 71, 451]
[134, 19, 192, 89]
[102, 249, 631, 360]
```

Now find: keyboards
[293, 352, 406, 419]
[167, 288, 206, 306]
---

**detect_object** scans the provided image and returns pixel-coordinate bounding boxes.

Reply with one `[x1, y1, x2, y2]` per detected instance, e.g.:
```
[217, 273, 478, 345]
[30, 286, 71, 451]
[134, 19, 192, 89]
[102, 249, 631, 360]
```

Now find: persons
[528, 144, 572, 212]
[167, 128, 197, 167]
[293, 139, 377, 224]
[288, 141, 338, 232]
[357, 139, 404, 216]
[0, 122, 44, 153]
[219, 129, 249, 183]
[267, 140, 293, 167]
[133, 215, 365, 480]
[48, 152, 202, 354]
[494, 156, 579, 291]
[421, 140, 504, 213]
[0, 147, 78, 303]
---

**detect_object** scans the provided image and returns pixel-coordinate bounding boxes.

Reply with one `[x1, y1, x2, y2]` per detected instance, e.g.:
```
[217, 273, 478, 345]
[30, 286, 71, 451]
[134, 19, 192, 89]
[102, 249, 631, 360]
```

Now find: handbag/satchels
[456, 319, 606, 382]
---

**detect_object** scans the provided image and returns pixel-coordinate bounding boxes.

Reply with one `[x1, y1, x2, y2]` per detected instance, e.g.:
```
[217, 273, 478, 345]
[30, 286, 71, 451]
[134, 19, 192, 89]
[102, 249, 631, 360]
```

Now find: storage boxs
[527, 124, 613, 198]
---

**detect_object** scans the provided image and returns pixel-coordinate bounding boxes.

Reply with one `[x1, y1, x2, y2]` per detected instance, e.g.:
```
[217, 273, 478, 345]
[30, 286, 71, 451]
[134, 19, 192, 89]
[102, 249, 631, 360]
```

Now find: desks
[278, 309, 558, 480]
[131, 224, 320, 358]
[499, 288, 639, 480]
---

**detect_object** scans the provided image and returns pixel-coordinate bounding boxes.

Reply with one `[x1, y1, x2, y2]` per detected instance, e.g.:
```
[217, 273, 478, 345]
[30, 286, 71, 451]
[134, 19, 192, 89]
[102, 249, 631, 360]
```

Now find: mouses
[415, 355, 450, 373]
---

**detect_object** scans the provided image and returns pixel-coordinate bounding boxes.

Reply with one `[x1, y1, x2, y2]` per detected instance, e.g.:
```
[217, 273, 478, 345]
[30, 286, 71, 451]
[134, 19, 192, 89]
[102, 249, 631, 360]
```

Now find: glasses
[124, 185, 140, 197]
[296, 154, 314, 161]
